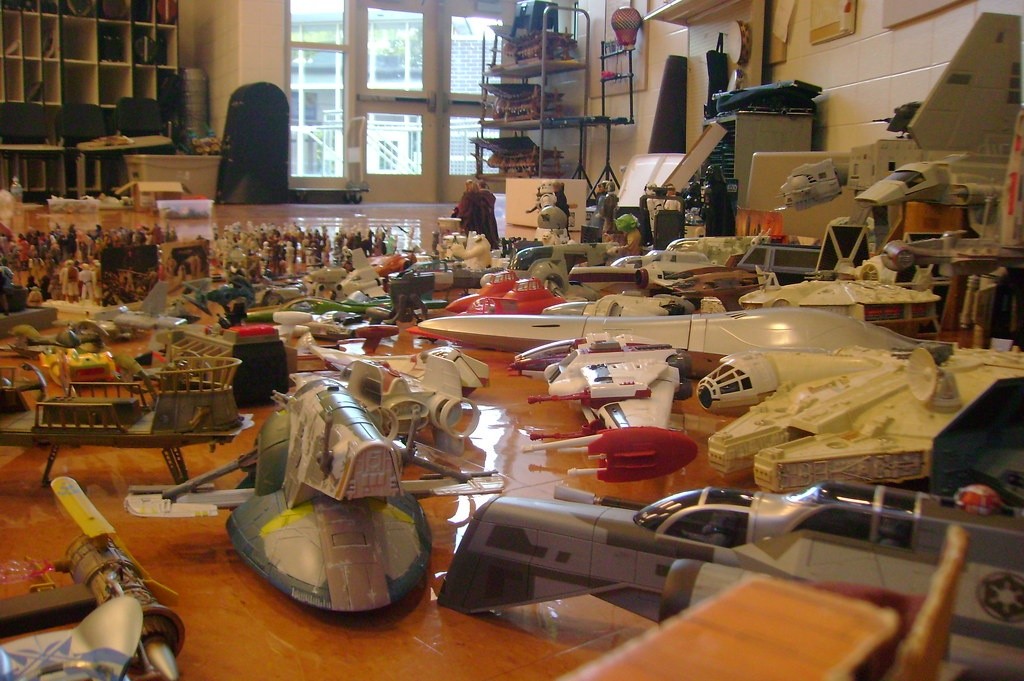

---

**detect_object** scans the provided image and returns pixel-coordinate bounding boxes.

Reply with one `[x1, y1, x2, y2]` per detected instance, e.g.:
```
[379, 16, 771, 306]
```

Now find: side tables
[700, 110, 815, 211]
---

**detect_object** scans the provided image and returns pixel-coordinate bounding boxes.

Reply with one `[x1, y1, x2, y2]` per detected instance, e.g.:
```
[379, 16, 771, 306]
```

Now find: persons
[0, 163, 737, 308]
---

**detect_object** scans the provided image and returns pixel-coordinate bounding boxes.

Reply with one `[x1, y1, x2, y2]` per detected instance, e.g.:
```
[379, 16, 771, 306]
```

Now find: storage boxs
[659, 121, 728, 194]
[114, 180, 193, 214]
[120, 154, 224, 207]
[46, 199, 102, 214]
[506, 177, 588, 232]
[156, 198, 215, 219]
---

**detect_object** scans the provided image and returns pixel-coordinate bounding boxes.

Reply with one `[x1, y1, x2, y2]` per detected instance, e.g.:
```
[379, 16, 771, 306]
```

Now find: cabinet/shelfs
[0, 0, 182, 203]
[474, 6, 592, 190]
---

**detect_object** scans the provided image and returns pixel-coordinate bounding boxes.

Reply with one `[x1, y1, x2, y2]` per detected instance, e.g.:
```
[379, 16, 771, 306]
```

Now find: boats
[123, 378, 445, 621]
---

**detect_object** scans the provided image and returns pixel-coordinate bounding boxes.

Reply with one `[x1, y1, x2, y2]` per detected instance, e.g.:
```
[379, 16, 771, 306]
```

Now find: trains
[437, 476, 1024, 678]
